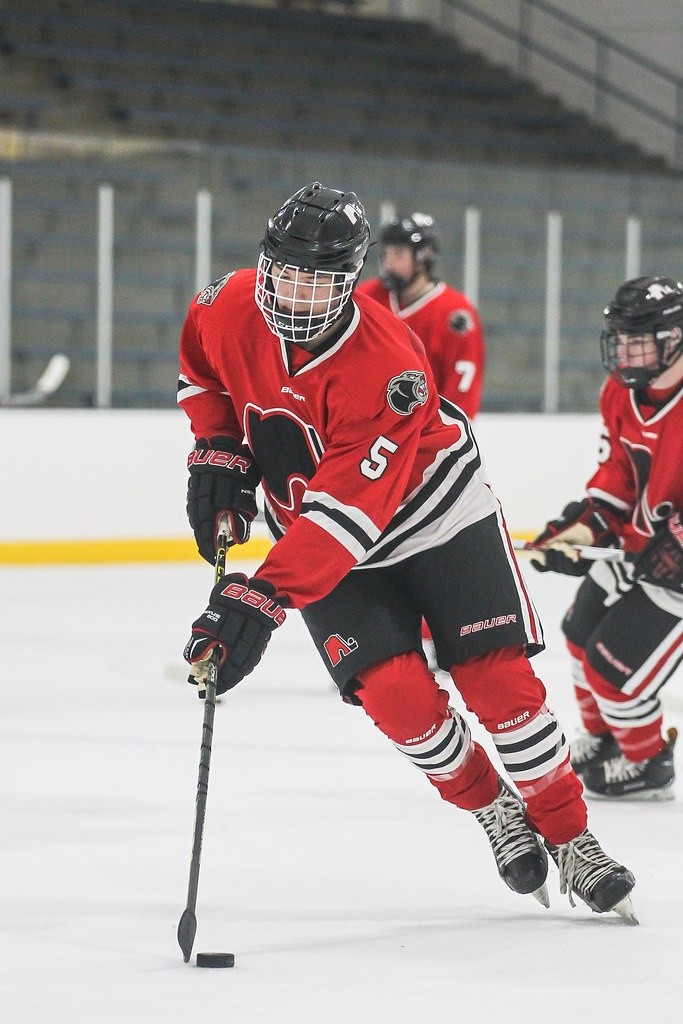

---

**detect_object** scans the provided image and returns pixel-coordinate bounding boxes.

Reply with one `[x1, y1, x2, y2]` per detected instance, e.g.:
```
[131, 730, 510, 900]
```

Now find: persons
[522, 275, 683, 803]
[356, 207, 486, 674]
[172, 184, 641, 926]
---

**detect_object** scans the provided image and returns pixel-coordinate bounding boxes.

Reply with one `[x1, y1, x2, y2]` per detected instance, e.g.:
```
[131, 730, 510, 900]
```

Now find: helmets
[378, 210, 439, 292]
[253, 183, 372, 343]
[602, 274, 683, 389]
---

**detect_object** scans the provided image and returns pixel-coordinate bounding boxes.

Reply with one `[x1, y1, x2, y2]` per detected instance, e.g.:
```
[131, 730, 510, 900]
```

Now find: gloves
[183, 573, 290, 700]
[632, 512, 683, 594]
[187, 435, 260, 565]
[524, 497, 624, 576]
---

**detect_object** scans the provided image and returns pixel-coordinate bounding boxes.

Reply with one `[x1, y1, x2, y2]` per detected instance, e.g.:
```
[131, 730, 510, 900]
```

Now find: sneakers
[540, 829, 641, 926]
[470, 775, 550, 909]
[570, 732, 620, 773]
[580, 727, 679, 801]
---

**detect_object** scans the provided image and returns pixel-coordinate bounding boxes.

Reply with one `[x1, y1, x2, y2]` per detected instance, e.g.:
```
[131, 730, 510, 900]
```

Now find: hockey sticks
[247, 516, 638, 565]
[174, 512, 239, 972]
